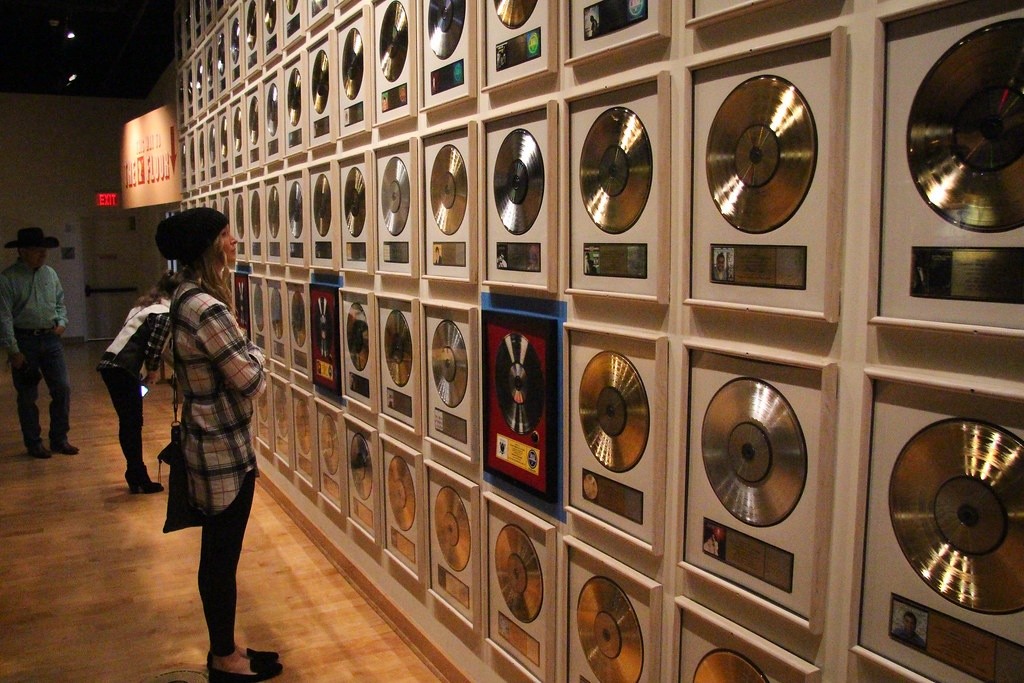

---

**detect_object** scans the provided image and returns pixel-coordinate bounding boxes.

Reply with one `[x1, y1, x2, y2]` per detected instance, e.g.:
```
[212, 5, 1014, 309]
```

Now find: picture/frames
[170, 1, 1023, 682]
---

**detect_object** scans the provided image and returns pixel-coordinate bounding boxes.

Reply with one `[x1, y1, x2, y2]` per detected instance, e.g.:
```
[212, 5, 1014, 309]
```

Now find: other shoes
[27, 444, 51, 458]
[50, 439, 79, 453]
[208, 659, 285, 683]
[207, 647, 279, 668]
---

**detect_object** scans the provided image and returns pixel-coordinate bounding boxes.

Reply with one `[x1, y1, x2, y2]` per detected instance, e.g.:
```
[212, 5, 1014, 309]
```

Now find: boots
[125, 461, 163, 494]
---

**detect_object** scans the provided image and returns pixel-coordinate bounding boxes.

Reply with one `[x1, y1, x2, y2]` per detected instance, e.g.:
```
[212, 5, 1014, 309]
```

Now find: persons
[156, 207, 283, 683]
[0, 226, 80, 459]
[99, 269, 181, 494]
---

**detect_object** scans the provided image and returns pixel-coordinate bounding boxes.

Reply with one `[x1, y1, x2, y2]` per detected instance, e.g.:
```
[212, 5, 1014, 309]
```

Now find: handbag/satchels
[158, 425, 206, 534]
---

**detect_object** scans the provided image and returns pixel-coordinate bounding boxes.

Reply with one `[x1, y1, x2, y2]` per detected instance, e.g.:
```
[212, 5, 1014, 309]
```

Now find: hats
[4, 227, 60, 249]
[155, 207, 229, 265]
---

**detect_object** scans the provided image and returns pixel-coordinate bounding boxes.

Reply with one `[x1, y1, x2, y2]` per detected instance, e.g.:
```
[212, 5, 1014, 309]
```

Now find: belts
[13, 326, 56, 336]
